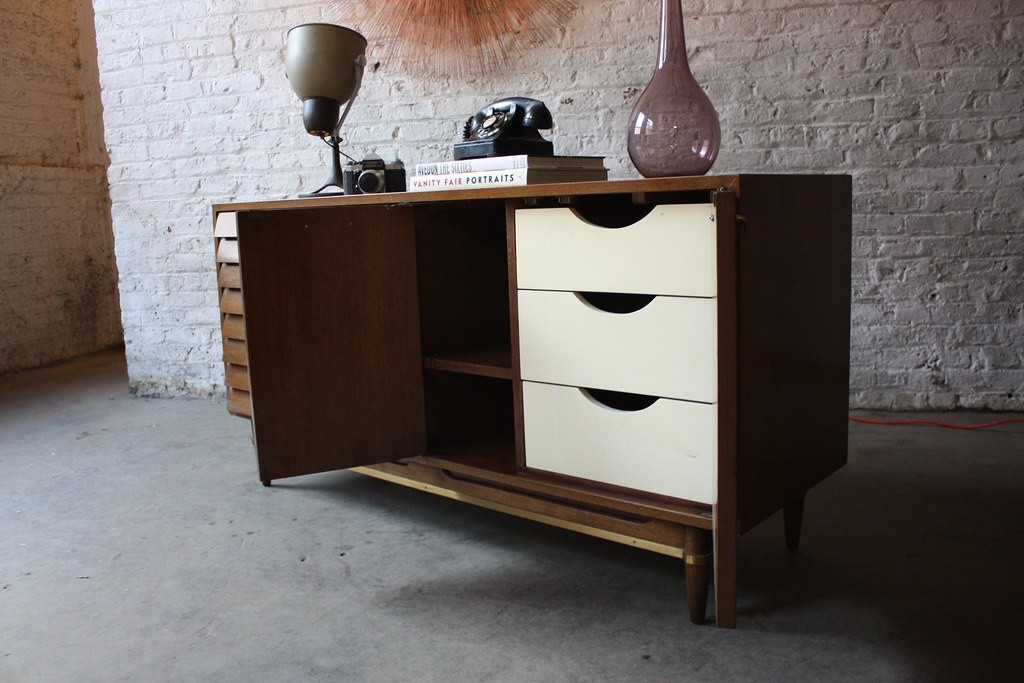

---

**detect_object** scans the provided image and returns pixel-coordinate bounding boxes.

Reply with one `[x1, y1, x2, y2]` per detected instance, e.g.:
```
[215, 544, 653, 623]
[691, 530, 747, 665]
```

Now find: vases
[627, 0, 721, 178]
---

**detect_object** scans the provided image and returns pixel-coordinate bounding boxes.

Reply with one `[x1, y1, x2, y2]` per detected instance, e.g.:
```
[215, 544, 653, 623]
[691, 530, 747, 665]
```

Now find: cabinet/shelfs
[210, 172, 850, 630]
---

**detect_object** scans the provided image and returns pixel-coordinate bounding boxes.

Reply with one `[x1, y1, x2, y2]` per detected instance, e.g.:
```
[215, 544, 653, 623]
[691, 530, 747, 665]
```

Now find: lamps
[285, 22, 368, 198]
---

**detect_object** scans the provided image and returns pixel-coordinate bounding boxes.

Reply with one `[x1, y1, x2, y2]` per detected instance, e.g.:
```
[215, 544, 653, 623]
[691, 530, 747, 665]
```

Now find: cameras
[343, 158, 406, 195]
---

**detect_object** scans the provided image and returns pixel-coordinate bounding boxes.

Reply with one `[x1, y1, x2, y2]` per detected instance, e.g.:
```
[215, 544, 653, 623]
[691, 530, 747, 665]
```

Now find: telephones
[453, 96, 553, 159]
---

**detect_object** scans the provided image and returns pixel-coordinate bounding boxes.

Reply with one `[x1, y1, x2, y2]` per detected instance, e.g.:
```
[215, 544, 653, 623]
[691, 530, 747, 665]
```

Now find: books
[409, 153, 610, 193]
